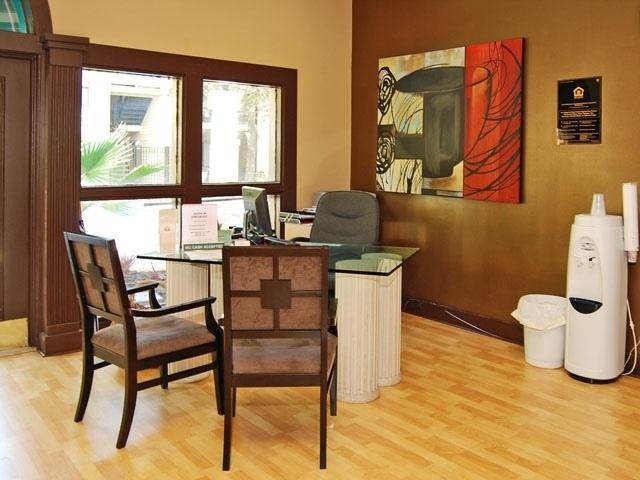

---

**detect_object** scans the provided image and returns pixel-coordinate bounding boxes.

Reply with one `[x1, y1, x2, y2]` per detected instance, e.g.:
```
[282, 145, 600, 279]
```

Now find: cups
[591, 193, 606, 218]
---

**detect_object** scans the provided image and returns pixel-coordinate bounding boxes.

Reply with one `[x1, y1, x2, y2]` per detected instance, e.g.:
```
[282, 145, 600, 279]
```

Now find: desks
[136, 240, 421, 404]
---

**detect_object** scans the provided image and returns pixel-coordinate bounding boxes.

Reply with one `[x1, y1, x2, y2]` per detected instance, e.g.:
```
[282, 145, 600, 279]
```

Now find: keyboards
[264, 234, 300, 247]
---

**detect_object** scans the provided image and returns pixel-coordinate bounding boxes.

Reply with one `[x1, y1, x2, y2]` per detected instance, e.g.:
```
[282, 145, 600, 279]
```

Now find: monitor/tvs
[241, 186, 277, 239]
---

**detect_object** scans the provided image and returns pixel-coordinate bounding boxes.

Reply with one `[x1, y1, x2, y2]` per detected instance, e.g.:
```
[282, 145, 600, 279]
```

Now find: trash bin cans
[517, 294, 567, 369]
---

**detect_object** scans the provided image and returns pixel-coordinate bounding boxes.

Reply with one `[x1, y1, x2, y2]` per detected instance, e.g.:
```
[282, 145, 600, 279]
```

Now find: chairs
[64, 231, 223, 448]
[220, 243, 339, 471]
[309, 190, 380, 245]
